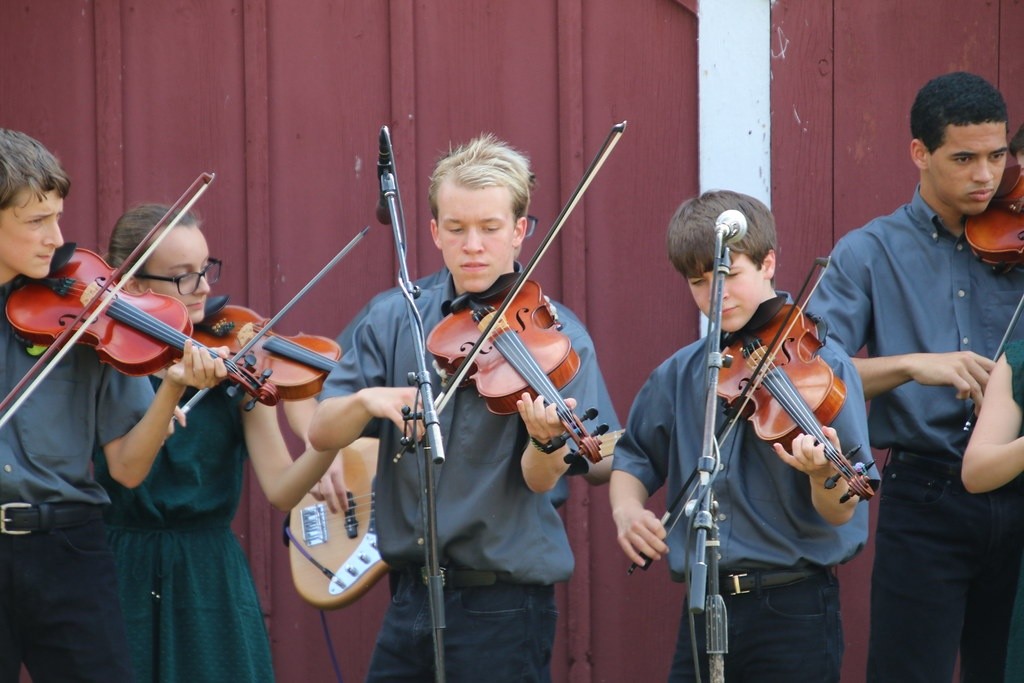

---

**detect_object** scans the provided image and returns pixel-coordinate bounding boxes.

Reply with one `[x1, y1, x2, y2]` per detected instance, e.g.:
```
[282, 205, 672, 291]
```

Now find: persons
[1010, 120, 1024, 176]
[93, 204, 339, 682]
[308, 133, 621, 682]
[803, 70, 1024, 683]
[0, 129, 229, 683]
[961, 337, 1022, 683]
[609, 188, 881, 681]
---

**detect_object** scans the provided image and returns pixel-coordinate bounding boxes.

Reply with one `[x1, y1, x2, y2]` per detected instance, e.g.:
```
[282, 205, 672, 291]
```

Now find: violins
[960, 162, 1024, 268]
[174, 299, 345, 411]
[427, 268, 609, 467]
[5, 248, 284, 412]
[711, 302, 881, 506]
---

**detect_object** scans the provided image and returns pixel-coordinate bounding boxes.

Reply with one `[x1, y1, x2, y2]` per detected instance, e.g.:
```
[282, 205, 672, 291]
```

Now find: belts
[0, 501, 102, 535]
[400, 565, 518, 588]
[719, 567, 820, 596]
[891, 448, 961, 479]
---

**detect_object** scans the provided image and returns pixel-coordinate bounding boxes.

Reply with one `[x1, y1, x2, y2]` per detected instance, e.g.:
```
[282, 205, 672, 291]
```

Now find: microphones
[715, 209, 747, 244]
[375, 126, 393, 225]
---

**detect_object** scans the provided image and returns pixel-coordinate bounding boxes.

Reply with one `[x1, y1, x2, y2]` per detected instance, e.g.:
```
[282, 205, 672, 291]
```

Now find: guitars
[284, 419, 631, 614]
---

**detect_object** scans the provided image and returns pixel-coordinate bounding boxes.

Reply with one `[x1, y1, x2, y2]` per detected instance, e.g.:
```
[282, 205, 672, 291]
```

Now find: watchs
[532, 438, 565, 454]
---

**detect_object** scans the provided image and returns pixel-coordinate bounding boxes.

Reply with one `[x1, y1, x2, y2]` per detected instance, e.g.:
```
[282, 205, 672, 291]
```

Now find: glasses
[525, 215, 538, 237]
[134, 256, 222, 295]
